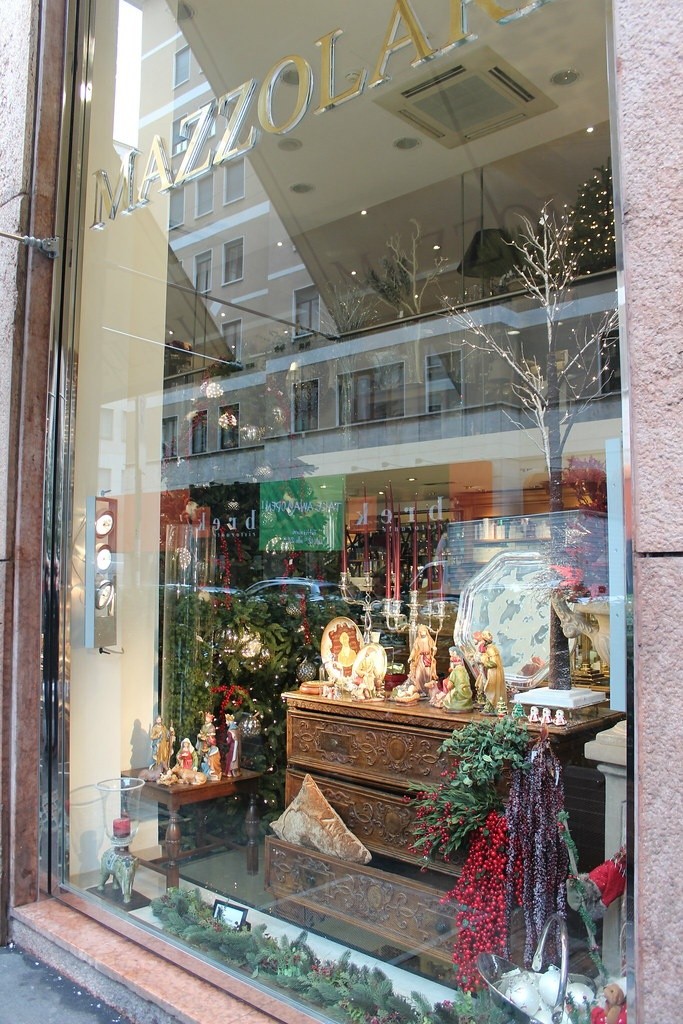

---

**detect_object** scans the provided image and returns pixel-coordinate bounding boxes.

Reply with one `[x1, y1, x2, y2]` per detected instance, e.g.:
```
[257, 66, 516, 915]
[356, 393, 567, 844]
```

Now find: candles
[384, 491, 392, 599]
[426, 512, 434, 600]
[341, 537, 347, 572]
[363, 487, 370, 573]
[390, 482, 397, 573]
[411, 500, 418, 590]
[438, 521, 445, 602]
[394, 503, 401, 600]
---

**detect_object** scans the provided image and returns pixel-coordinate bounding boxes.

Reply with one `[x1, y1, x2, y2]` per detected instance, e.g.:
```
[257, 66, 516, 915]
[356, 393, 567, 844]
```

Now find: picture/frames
[212, 900, 249, 932]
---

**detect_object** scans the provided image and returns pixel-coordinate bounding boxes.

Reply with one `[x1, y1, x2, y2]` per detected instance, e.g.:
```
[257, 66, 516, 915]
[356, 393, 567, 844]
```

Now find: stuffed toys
[590, 984, 627, 1024]
[566, 844, 628, 923]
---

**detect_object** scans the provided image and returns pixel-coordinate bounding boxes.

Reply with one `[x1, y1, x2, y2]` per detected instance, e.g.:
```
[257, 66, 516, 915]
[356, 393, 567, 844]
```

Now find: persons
[471, 631, 497, 706]
[443, 646, 466, 697]
[138, 716, 176, 781]
[222, 714, 243, 778]
[475, 632, 509, 713]
[200, 736, 223, 781]
[353, 647, 382, 697]
[407, 623, 438, 697]
[175, 738, 199, 772]
[196, 712, 216, 769]
[444, 657, 473, 711]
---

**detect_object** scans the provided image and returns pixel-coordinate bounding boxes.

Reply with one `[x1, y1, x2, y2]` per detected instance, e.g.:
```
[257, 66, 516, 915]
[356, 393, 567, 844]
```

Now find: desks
[120, 761, 264, 894]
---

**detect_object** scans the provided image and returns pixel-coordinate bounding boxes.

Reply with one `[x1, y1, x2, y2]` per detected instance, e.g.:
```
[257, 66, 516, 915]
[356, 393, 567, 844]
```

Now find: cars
[198, 585, 244, 598]
[242, 576, 363, 613]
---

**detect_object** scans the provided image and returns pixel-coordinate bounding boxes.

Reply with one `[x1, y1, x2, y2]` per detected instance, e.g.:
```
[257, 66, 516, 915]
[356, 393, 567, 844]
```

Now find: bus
[406, 511, 611, 704]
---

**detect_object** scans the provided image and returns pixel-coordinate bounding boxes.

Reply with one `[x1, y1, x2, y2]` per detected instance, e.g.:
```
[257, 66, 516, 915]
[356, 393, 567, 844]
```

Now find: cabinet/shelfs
[264, 690, 603, 988]
[584, 718, 627, 985]
[456, 487, 592, 582]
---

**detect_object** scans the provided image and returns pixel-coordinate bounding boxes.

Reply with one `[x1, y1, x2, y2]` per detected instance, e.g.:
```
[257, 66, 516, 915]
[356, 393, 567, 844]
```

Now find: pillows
[269, 774, 372, 866]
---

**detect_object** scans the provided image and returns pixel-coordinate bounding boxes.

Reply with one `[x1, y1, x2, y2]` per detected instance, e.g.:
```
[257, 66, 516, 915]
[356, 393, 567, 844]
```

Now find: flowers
[547, 468, 609, 603]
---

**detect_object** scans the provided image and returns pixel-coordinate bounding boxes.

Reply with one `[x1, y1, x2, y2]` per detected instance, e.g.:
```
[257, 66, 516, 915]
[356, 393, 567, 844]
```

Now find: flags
[260, 475, 348, 553]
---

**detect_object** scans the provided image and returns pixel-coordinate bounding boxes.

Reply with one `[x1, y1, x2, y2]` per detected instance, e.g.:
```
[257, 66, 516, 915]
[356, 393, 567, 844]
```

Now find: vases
[505, 278, 541, 313]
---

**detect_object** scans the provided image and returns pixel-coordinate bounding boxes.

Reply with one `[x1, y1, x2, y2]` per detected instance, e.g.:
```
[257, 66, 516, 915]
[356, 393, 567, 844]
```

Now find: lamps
[453, 228, 527, 308]
